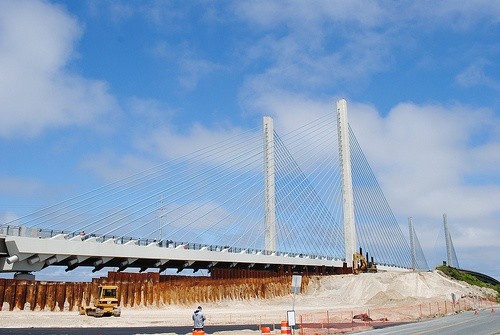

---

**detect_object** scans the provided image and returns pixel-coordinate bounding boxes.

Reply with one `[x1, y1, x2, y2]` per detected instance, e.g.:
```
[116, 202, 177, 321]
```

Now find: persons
[193, 305, 205, 331]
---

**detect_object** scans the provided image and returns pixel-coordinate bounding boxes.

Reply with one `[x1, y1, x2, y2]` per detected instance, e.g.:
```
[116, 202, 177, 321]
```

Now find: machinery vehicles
[79, 285, 121, 318]
[352, 251, 377, 275]
